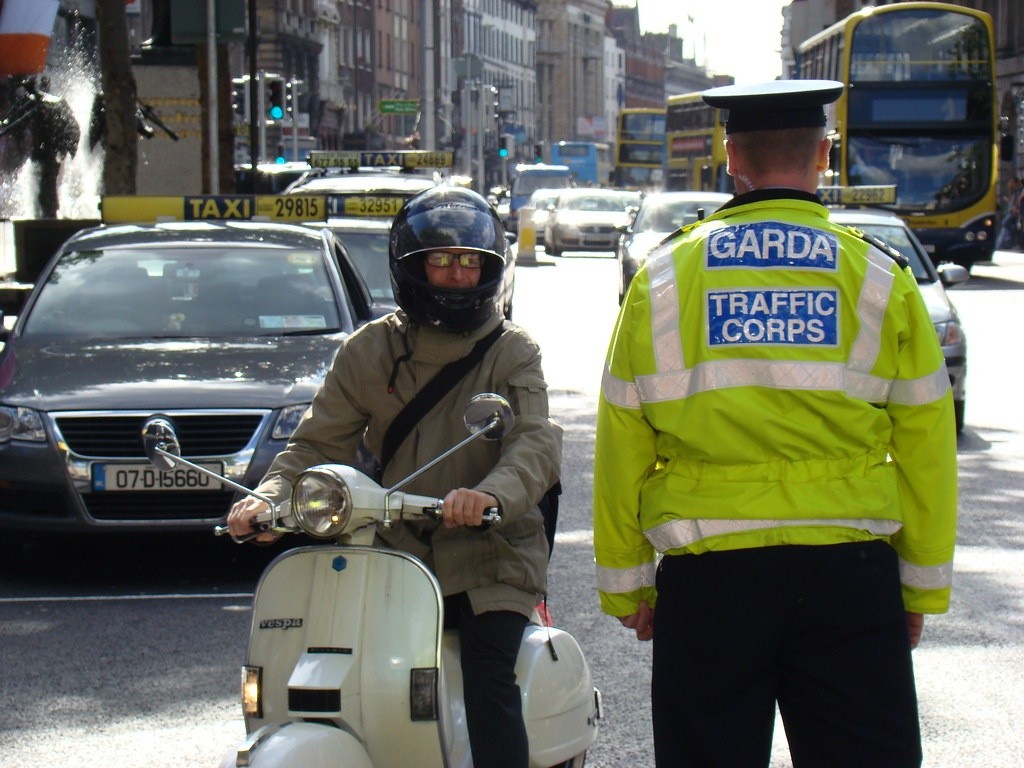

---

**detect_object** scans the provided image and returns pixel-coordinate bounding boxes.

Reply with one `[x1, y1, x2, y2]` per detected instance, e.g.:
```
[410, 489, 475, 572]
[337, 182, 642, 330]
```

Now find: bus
[662, 85, 737, 193]
[792, 1, 1016, 264]
[552, 141, 609, 188]
[612, 106, 666, 190]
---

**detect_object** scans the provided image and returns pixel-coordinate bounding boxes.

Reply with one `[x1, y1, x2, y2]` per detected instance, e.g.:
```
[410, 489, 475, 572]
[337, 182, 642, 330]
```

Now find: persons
[593, 78, 958, 768]
[219, 187, 564, 768]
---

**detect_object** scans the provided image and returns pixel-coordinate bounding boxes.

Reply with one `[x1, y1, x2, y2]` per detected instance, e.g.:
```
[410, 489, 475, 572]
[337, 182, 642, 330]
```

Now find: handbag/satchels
[538, 480, 562, 561]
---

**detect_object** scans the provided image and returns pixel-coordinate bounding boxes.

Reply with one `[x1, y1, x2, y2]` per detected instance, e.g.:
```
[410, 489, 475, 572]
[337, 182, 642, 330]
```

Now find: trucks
[504, 161, 575, 236]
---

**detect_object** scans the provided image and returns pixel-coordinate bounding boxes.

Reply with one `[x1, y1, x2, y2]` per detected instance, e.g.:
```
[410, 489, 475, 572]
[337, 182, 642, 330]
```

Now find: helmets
[388, 186, 506, 333]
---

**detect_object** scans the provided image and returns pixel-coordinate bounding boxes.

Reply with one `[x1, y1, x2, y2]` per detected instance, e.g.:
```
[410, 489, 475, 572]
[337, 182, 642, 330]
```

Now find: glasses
[424, 250, 486, 269]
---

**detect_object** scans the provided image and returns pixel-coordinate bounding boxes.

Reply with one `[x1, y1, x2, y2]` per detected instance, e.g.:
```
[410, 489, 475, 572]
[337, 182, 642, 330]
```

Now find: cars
[525, 186, 642, 258]
[811, 181, 970, 437]
[616, 193, 735, 306]
[0, 187, 389, 564]
[263, 146, 456, 325]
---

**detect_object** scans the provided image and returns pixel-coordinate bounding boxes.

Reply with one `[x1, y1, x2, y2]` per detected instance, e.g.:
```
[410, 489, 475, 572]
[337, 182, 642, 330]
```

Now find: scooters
[142, 392, 606, 768]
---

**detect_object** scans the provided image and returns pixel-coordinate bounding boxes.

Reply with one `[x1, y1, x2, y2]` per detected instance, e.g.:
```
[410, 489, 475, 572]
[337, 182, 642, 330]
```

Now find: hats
[702, 80, 845, 134]
